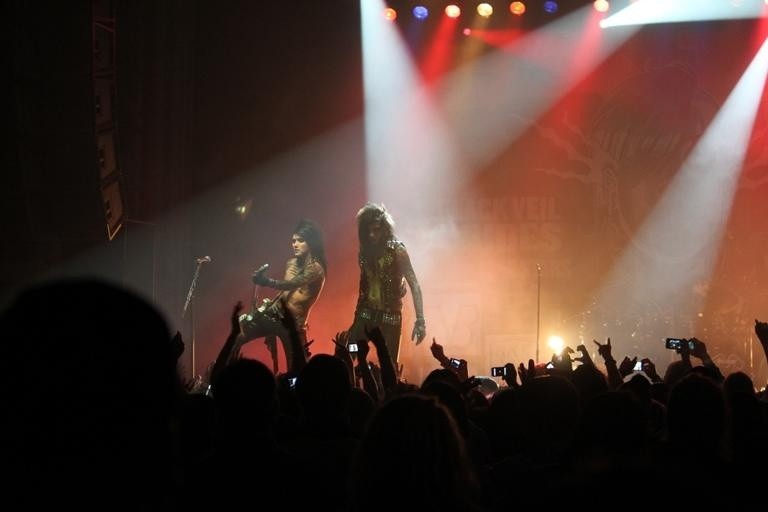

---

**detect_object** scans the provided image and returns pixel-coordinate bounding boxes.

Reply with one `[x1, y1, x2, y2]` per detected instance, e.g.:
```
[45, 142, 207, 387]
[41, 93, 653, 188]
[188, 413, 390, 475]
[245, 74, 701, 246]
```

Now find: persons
[348, 205, 425, 368]
[233, 219, 326, 372]
[1, 279, 767, 511]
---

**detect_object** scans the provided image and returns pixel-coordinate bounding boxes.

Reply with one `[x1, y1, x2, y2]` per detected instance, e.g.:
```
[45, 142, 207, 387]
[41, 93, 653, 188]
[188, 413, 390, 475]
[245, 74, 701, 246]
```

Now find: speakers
[14, 0, 129, 245]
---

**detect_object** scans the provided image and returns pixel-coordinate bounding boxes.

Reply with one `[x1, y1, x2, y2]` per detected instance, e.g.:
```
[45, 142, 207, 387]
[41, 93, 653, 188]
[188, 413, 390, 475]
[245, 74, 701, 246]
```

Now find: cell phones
[688, 340, 694, 350]
[666, 338, 681, 349]
[492, 367, 507, 377]
[545, 362, 555, 369]
[450, 358, 461, 369]
[632, 362, 642, 371]
[348, 343, 359, 352]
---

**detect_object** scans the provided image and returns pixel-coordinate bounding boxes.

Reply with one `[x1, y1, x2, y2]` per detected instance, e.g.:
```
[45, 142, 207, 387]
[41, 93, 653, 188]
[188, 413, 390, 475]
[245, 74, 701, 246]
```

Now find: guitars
[238, 263, 272, 330]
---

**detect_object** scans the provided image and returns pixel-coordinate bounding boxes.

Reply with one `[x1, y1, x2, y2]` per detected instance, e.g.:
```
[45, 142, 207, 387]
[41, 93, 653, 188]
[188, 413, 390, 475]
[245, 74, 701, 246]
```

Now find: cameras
[287, 377, 298, 388]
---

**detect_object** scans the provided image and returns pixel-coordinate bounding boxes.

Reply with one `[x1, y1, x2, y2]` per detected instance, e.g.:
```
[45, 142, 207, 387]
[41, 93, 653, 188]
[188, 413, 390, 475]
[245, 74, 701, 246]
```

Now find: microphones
[256, 264, 271, 273]
[197, 255, 211, 265]
